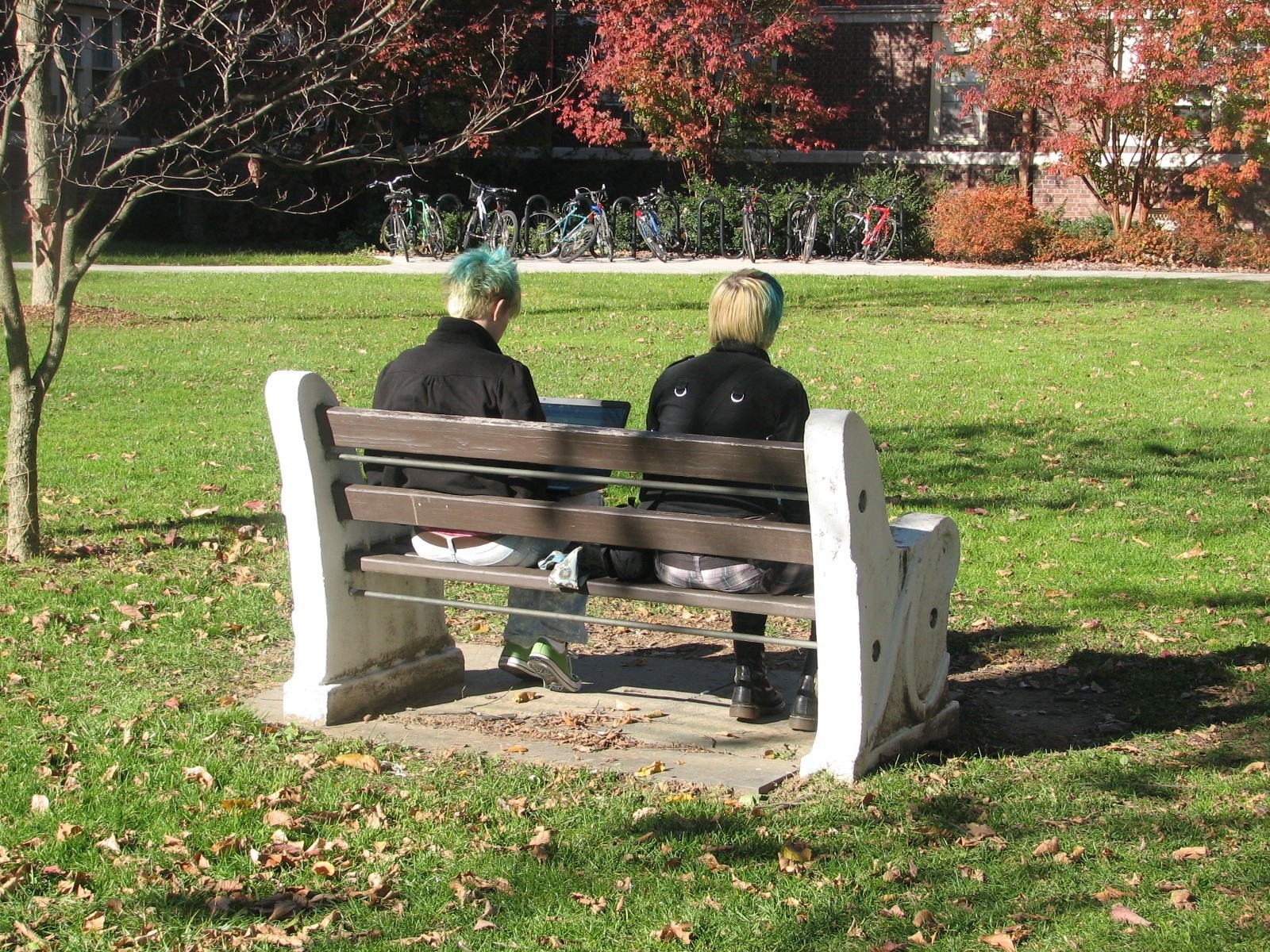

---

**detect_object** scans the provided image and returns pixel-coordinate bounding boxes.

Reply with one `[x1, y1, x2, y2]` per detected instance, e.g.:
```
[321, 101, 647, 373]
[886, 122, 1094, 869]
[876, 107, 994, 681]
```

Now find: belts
[415, 524, 501, 551]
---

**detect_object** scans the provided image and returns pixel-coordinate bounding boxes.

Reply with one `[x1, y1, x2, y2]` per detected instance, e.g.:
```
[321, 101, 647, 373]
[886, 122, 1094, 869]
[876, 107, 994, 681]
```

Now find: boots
[729, 612, 785, 720]
[790, 622, 819, 733]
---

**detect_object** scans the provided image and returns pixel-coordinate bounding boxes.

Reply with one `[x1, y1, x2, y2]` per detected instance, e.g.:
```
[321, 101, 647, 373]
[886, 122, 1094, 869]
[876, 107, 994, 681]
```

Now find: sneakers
[499, 639, 541, 680]
[528, 638, 581, 693]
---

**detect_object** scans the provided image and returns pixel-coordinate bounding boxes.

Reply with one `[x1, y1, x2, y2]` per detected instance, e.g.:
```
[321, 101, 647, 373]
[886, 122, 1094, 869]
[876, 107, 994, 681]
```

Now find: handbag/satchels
[601, 496, 650, 580]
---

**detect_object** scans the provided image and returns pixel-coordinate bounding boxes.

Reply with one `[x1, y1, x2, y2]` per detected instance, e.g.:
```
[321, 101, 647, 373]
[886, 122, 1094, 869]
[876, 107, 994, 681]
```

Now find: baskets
[470, 184, 494, 204]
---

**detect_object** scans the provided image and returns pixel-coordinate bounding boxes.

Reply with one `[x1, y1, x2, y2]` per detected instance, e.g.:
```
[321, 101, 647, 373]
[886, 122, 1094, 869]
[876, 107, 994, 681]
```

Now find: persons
[364, 241, 584, 693]
[639, 267, 817, 732]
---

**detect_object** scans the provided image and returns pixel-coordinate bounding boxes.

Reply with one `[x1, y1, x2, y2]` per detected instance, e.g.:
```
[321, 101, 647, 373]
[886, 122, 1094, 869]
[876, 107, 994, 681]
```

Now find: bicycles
[827, 193, 897, 265]
[403, 187, 445, 261]
[365, 174, 413, 263]
[788, 191, 825, 264]
[730, 186, 765, 264]
[518, 183, 614, 264]
[628, 186, 689, 264]
[455, 172, 519, 257]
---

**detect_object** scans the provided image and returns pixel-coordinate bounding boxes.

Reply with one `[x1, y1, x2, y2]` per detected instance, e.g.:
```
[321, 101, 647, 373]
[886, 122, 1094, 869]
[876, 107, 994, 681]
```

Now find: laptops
[536, 397, 631, 493]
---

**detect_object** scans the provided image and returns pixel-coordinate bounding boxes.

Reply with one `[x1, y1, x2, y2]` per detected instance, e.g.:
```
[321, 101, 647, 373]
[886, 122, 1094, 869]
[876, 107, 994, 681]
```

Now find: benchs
[265, 371, 961, 787]
